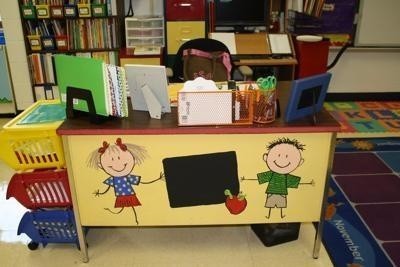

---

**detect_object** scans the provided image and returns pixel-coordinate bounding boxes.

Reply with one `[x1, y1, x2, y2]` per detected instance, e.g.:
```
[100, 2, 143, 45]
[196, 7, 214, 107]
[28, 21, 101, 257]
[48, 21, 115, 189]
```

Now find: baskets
[177, 90, 254, 126]
[1, 100, 89, 251]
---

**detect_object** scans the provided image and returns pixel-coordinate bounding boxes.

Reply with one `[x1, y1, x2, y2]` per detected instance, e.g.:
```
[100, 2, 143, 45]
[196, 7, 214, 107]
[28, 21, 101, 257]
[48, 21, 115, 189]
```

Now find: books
[18, 0, 129, 119]
[287, 0, 325, 28]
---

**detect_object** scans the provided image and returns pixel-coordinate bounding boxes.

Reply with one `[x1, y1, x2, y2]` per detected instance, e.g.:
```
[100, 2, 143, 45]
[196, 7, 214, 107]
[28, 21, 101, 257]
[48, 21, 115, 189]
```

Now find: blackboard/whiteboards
[352, 0, 399, 48]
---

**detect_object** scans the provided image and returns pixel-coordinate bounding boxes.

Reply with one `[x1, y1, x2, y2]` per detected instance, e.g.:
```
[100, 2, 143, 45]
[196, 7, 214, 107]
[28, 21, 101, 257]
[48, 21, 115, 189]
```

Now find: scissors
[257, 76, 277, 123]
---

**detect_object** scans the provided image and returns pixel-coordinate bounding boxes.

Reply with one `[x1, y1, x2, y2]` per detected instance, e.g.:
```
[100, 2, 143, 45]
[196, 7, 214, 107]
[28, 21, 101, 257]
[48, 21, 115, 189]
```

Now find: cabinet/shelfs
[18, 0, 125, 101]
[126, 15, 166, 48]
[167, 21, 206, 55]
[166, 0, 205, 21]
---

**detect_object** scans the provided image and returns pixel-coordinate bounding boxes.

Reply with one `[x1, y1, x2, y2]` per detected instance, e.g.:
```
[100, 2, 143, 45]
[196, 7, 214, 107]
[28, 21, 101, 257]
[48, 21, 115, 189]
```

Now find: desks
[56, 99, 341, 263]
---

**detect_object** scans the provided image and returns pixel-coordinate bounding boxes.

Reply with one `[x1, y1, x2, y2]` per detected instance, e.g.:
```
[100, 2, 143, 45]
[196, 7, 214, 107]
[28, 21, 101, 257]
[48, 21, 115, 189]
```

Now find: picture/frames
[124, 64, 171, 119]
[283, 72, 332, 123]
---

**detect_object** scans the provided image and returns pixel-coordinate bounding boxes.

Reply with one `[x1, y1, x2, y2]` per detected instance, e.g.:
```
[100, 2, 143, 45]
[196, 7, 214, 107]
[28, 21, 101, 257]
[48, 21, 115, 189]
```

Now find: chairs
[167, 35, 254, 84]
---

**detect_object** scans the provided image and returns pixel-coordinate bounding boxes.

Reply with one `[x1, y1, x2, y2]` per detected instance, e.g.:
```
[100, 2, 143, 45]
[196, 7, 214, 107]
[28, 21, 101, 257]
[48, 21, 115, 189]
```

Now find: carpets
[311, 102, 400, 267]
